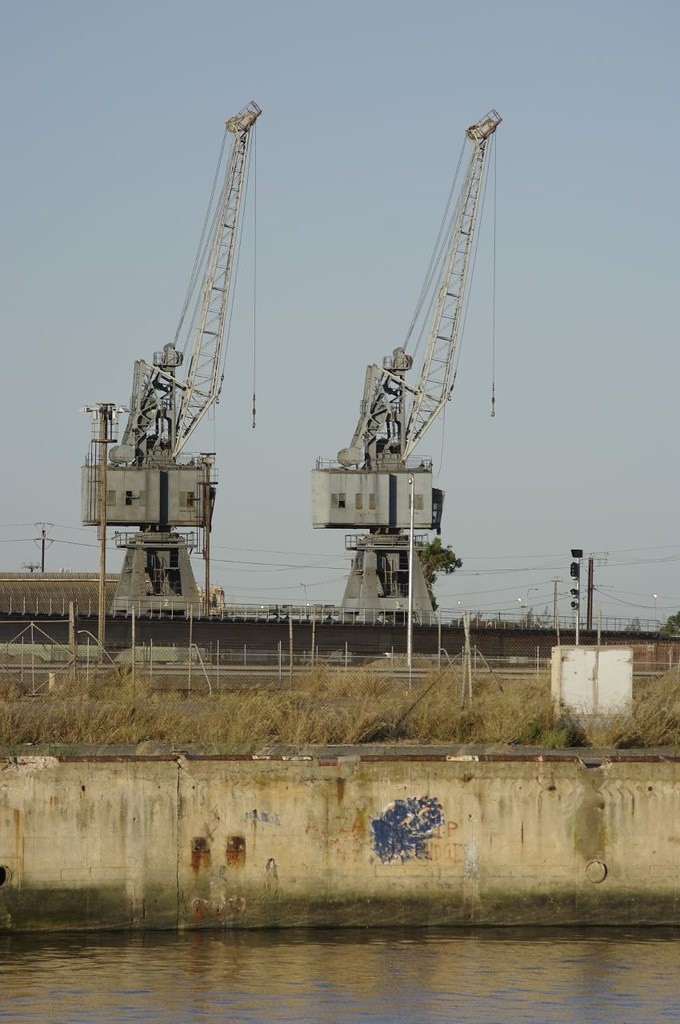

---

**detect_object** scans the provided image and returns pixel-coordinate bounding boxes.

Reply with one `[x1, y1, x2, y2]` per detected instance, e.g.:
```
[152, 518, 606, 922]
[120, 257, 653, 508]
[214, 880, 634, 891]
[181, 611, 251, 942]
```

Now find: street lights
[526, 587, 540, 611]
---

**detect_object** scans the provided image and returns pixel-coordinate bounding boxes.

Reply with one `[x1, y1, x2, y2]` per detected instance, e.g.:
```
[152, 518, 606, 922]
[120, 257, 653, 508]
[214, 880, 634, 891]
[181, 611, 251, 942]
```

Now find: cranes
[81, 101, 263, 621]
[308, 108, 505, 626]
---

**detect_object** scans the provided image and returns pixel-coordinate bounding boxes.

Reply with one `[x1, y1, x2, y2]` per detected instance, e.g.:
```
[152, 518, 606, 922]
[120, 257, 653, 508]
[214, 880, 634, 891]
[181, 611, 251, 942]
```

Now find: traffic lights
[570, 589, 577, 595]
[570, 601, 576, 609]
[570, 562, 579, 580]
